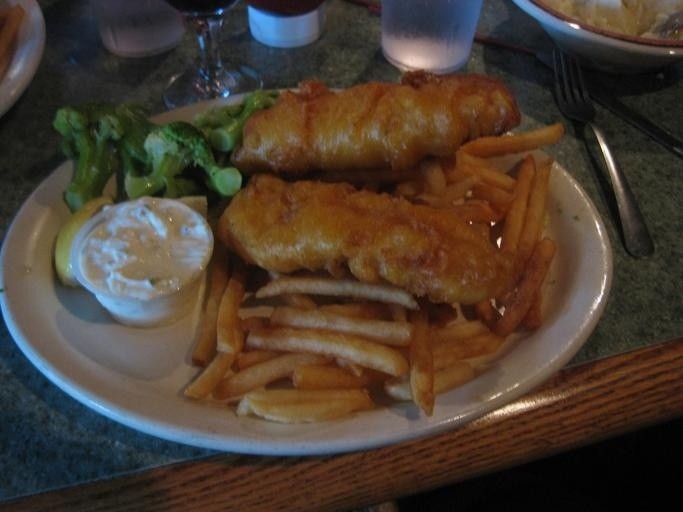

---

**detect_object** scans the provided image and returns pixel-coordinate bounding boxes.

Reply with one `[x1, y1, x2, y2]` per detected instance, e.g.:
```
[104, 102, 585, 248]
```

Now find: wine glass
[160, 0, 263, 110]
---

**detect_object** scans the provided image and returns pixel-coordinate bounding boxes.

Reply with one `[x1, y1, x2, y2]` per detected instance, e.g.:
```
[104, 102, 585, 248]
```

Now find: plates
[0, 0, 45, 120]
[0, 86, 616, 458]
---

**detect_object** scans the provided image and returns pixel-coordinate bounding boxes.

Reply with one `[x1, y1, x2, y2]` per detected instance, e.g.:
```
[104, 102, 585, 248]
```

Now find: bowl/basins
[511, 0, 683, 70]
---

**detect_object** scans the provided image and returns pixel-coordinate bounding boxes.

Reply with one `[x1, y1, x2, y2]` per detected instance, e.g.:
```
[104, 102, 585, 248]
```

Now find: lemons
[53, 195, 113, 288]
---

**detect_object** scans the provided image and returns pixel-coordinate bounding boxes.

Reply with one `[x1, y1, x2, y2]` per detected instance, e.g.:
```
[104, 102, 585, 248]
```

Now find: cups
[376, 1, 485, 75]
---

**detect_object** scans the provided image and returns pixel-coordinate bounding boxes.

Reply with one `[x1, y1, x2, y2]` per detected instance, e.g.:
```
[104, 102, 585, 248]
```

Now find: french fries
[183, 121, 566, 423]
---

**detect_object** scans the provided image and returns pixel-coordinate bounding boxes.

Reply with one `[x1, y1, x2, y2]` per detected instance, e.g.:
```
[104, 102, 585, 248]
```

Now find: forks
[550, 48, 655, 261]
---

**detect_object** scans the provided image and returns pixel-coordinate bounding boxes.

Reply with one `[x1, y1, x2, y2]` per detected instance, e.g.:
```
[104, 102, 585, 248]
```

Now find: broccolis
[53, 88, 280, 214]
